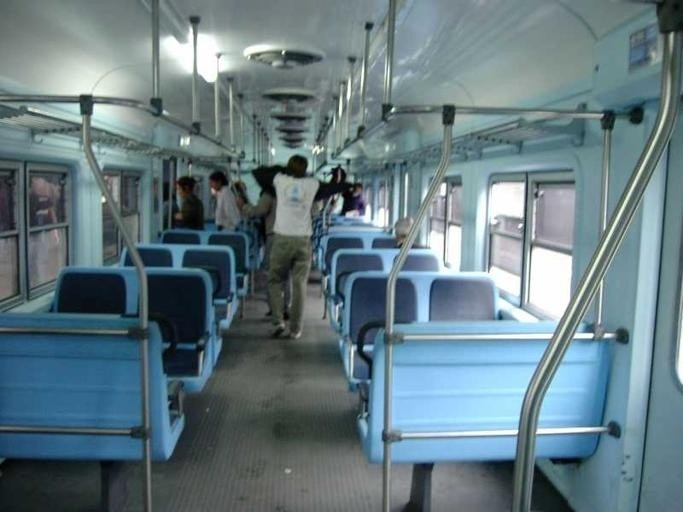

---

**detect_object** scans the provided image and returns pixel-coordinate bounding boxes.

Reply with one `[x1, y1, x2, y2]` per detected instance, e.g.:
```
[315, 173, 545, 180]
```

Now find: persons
[173, 154, 367, 339]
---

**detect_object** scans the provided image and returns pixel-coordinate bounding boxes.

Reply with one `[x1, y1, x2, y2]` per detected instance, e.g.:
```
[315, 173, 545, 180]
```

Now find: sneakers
[272, 323, 287, 337]
[290, 330, 303, 338]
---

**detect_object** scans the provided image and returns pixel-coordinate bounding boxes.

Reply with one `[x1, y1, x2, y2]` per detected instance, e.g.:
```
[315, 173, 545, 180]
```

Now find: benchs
[0, 313, 185, 511]
[339, 267, 499, 390]
[53, 265, 213, 398]
[121, 218, 267, 318]
[360, 319, 613, 512]
[312, 213, 422, 248]
[330, 244, 444, 331]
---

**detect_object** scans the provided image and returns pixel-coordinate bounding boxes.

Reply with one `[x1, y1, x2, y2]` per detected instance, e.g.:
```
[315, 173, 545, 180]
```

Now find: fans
[261, 88, 315, 106]
[283, 143, 302, 148]
[271, 111, 309, 123]
[280, 136, 306, 142]
[241, 45, 326, 71]
[274, 125, 307, 135]
